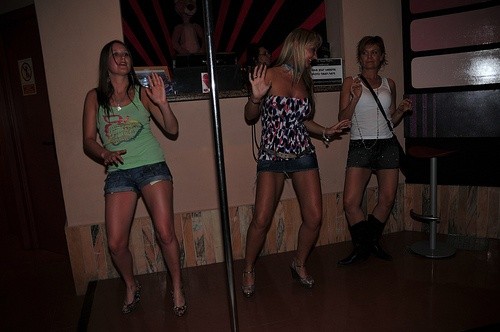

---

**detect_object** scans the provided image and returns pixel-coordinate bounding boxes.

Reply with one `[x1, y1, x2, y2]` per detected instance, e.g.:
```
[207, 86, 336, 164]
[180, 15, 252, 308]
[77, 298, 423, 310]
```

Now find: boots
[338, 220, 369, 265]
[367, 214, 394, 260]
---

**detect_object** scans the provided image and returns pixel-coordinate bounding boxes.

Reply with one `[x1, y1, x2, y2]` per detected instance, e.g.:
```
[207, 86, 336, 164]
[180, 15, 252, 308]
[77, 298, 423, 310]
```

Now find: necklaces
[354, 74, 379, 148]
[283, 63, 293, 75]
[112, 96, 123, 111]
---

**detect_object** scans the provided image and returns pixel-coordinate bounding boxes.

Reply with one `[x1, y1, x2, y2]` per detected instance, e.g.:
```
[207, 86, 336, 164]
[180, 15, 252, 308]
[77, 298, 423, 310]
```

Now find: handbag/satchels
[392, 133, 406, 165]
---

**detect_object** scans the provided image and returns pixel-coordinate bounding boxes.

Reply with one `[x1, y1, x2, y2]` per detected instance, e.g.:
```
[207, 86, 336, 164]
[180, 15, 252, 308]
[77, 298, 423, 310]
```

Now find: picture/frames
[130, 66, 171, 88]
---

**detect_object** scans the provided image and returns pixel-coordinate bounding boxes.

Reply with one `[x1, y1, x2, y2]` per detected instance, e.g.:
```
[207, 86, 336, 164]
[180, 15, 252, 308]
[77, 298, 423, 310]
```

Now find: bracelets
[323, 128, 335, 142]
[250, 98, 260, 104]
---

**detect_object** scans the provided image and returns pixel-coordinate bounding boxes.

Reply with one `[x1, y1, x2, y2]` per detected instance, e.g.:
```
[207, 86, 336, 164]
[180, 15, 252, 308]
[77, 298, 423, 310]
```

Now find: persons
[242, 27, 352, 297]
[240, 44, 268, 88]
[82, 39, 187, 316]
[337, 34, 412, 265]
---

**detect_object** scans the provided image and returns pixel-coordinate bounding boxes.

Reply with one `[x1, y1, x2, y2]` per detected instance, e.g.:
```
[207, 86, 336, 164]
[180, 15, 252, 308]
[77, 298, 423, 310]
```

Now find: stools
[407, 147, 458, 258]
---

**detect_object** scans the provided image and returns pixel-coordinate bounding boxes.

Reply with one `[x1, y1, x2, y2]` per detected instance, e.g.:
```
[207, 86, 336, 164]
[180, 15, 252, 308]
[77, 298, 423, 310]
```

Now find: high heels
[241, 264, 256, 297]
[171, 286, 187, 317]
[290, 257, 316, 289]
[121, 280, 144, 313]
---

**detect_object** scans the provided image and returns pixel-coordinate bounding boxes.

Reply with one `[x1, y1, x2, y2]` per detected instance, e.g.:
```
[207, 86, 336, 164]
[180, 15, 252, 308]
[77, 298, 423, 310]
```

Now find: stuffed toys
[171, 0, 206, 55]
